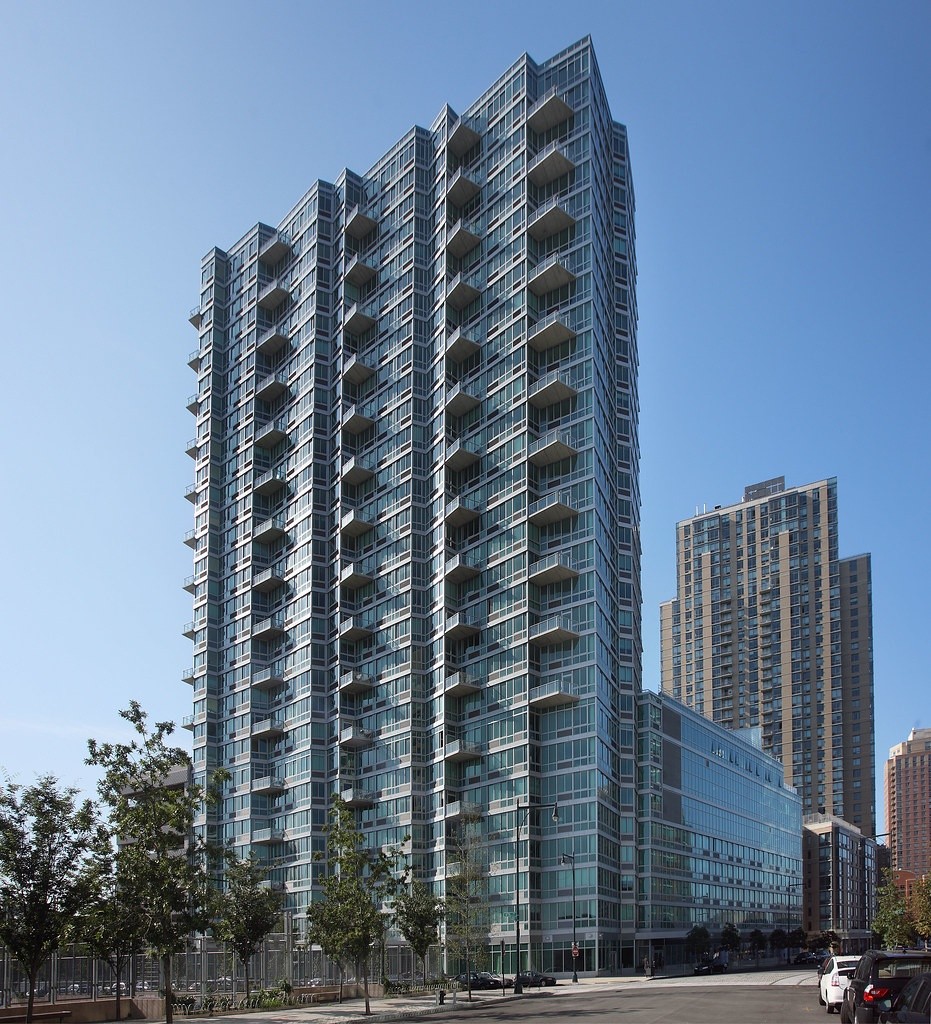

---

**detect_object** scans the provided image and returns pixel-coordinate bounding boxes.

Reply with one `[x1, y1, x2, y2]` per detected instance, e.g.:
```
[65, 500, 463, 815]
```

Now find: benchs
[0, 1010, 72, 1024]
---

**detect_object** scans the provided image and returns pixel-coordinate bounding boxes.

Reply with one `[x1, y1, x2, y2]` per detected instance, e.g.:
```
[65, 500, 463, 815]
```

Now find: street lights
[786, 882, 808, 964]
[560, 851, 577, 982]
[513, 797, 558, 995]
[857, 828, 894, 881]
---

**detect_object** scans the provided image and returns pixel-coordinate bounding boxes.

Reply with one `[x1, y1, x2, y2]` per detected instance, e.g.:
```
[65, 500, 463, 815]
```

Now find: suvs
[840, 946, 931, 1024]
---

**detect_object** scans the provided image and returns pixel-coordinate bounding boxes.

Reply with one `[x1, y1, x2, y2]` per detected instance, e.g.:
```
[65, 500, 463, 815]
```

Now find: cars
[877, 971, 931, 1024]
[307, 976, 365, 986]
[816, 949, 831, 965]
[694, 959, 729, 976]
[817, 955, 864, 1013]
[448, 971, 501, 990]
[513, 970, 556, 987]
[187, 975, 239, 991]
[794, 952, 816, 965]
[470, 971, 514, 988]
[25, 980, 160, 998]
[818, 953, 846, 988]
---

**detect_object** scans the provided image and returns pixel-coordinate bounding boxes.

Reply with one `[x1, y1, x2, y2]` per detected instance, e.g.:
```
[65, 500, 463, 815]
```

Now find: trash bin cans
[644, 965, 655, 977]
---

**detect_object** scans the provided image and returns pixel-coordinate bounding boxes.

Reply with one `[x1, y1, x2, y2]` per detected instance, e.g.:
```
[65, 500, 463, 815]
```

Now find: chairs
[879, 970, 893, 979]
